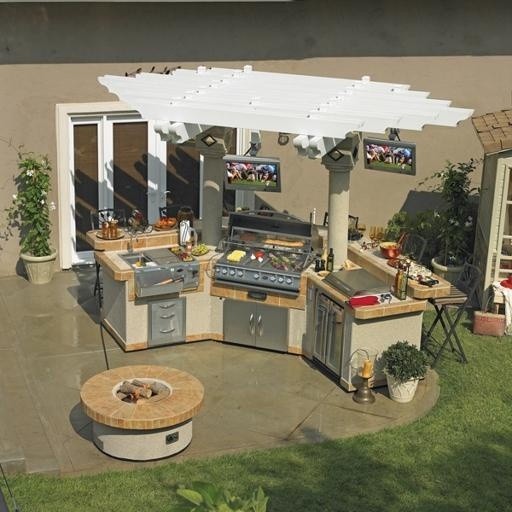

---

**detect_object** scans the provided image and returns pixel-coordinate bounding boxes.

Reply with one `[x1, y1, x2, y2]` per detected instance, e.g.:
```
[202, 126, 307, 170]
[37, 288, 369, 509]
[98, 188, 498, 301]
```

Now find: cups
[180, 219, 198, 246]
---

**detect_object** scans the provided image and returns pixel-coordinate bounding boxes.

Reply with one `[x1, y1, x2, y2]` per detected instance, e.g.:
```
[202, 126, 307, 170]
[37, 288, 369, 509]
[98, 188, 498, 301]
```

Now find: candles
[343, 347, 373, 378]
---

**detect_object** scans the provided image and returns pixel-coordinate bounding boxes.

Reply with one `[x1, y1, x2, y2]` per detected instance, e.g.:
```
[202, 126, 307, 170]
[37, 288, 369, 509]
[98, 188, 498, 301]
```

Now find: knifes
[152, 275, 184, 286]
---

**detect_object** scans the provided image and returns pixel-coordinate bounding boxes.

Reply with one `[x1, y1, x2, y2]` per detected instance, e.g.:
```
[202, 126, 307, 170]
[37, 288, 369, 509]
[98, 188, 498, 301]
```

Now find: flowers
[419, 157, 481, 266]
[0, 139, 57, 257]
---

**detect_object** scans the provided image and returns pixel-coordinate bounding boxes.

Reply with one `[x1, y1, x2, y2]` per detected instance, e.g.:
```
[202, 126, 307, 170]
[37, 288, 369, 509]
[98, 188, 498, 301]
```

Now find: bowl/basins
[380, 241, 402, 259]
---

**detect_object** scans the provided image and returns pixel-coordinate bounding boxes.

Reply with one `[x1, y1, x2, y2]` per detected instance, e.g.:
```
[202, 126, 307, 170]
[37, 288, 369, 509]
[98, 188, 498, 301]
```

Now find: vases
[20, 247, 57, 284]
[432, 254, 470, 285]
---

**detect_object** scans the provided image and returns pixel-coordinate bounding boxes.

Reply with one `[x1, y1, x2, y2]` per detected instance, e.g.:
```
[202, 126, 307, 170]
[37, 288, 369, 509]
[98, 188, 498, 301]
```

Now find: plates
[96, 233, 125, 240]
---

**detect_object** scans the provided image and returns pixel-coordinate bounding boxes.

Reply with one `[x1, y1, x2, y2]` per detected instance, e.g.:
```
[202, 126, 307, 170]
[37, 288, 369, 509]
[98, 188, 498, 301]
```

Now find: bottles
[311, 208, 318, 225]
[315, 248, 334, 272]
[101, 221, 118, 237]
[395, 269, 408, 300]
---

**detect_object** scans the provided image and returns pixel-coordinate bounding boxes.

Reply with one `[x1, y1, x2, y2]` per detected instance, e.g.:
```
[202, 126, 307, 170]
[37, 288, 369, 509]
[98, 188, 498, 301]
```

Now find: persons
[228, 164, 275, 185]
[367, 144, 412, 169]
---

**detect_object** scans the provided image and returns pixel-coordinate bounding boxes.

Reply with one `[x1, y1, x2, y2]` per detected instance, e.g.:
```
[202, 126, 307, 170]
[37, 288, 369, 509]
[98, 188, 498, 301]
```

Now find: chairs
[257, 205, 273, 217]
[159, 204, 182, 218]
[323, 213, 358, 230]
[422, 265, 482, 369]
[90, 209, 127, 308]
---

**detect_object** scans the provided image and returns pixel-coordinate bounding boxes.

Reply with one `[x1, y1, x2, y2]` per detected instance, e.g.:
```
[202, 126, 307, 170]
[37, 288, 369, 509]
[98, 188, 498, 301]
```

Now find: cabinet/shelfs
[86, 225, 452, 392]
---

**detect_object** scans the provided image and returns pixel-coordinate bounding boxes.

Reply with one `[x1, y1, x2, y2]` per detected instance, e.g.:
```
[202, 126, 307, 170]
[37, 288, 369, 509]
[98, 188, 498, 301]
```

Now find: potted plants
[382, 341, 426, 403]
